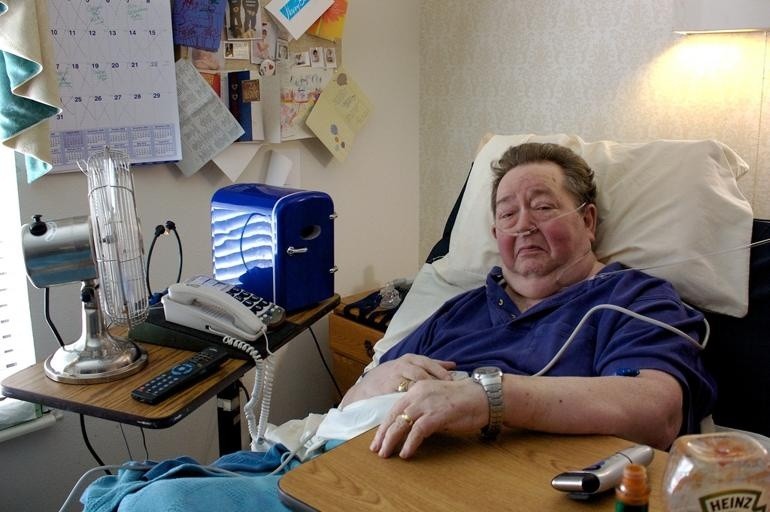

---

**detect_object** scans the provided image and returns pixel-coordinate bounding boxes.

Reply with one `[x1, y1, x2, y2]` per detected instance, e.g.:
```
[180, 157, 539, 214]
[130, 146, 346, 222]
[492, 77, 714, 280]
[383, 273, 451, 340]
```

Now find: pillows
[447, 133, 759, 319]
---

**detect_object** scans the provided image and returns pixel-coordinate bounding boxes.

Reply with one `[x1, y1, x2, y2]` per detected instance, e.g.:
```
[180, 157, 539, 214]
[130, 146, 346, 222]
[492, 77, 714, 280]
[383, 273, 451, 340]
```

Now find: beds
[76, 130, 753, 512]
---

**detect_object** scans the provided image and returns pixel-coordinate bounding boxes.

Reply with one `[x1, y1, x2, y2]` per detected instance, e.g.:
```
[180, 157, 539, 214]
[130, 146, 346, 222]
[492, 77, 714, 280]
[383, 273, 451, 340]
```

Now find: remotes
[131, 342, 229, 405]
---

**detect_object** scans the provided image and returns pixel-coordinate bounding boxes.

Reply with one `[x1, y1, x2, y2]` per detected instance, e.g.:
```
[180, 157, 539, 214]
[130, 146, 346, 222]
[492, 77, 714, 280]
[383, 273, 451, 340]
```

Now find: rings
[397, 378, 410, 393]
[400, 411, 413, 427]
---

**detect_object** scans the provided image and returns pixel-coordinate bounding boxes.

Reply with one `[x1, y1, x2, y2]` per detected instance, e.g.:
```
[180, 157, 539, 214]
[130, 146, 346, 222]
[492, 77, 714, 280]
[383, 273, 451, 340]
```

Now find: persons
[337, 142, 716, 459]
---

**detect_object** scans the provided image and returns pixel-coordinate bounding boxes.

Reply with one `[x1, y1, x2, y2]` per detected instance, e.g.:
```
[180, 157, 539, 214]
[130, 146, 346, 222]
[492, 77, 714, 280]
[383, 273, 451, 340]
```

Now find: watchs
[470, 365, 505, 442]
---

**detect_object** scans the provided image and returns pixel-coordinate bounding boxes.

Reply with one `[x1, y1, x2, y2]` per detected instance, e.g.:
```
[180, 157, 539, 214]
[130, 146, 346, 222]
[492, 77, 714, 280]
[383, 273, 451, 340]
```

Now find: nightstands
[324, 282, 411, 405]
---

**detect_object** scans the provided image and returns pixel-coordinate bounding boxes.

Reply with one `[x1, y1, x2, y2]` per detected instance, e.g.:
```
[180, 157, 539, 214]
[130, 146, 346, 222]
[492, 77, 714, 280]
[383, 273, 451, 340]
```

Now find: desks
[270, 410, 675, 512]
[0, 291, 343, 462]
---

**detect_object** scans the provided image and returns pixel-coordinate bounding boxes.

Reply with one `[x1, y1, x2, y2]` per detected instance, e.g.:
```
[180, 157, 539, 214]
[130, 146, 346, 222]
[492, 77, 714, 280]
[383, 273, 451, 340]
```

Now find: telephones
[161, 275, 284, 341]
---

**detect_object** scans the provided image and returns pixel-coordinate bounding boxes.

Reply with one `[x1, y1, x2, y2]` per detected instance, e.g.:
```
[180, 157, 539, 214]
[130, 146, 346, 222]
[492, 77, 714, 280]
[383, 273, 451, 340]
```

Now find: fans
[19, 149, 155, 386]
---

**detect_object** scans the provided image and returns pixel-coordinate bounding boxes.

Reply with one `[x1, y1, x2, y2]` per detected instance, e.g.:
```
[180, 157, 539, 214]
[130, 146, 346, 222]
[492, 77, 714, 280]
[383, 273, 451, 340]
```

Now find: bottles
[659, 432, 769, 512]
[615, 464, 652, 510]
